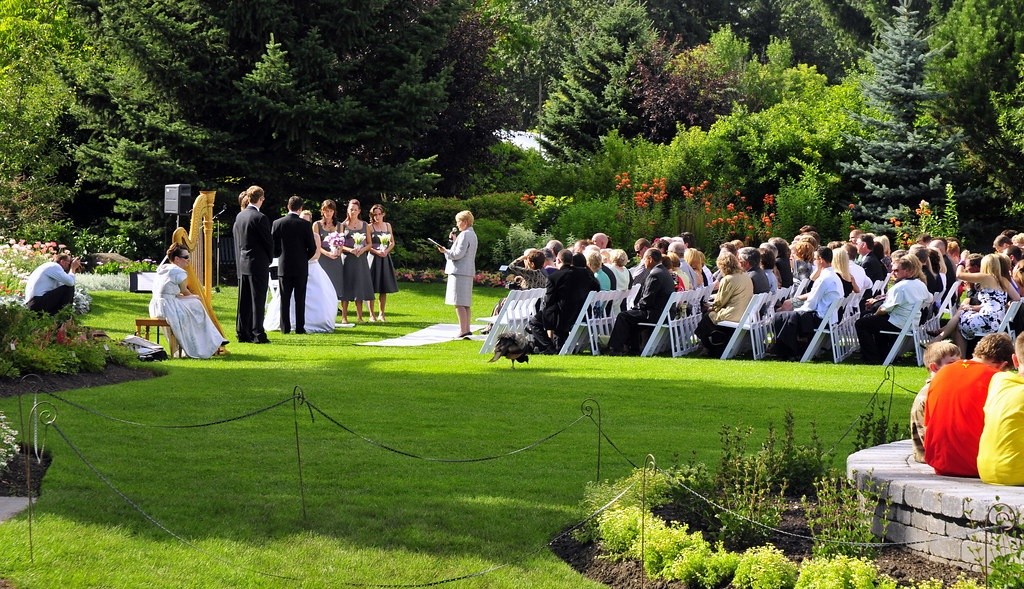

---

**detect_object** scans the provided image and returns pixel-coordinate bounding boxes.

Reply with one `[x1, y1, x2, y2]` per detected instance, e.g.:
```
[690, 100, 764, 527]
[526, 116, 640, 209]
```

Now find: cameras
[70, 258, 88, 269]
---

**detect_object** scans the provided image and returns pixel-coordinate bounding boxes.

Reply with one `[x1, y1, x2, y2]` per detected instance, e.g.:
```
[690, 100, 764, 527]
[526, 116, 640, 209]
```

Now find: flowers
[351, 232, 366, 251]
[323, 231, 345, 257]
[377, 233, 391, 251]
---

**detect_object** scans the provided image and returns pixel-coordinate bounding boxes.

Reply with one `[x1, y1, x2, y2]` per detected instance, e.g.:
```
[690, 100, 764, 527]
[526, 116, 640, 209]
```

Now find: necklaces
[354, 227, 356, 229]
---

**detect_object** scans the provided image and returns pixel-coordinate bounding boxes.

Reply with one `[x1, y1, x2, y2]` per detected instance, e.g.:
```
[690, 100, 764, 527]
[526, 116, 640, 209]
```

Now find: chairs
[475, 279, 1023, 367]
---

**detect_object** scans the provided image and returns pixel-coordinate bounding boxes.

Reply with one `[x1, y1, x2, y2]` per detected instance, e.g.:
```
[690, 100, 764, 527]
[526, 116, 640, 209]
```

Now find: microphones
[449, 228, 458, 242]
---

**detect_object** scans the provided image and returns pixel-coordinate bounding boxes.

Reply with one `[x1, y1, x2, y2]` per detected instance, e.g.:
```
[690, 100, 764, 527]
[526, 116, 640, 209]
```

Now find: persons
[481, 224, 1024, 365]
[365, 204, 399, 321]
[25, 253, 80, 320]
[909, 342, 962, 463]
[313, 199, 345, 299]
[976, 330, 1024, 488]
[437, 210, 478, 338]
[149, 242, 230, 359]
[924, 332, 1015, 477]
[232, 186, 273, 343]
[263, 209, 339, 333]
[271, 196, 317, 334]
[341, 200, 376, 324]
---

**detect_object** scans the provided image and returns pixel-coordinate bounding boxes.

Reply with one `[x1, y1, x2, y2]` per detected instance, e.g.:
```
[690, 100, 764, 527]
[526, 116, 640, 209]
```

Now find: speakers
[164, 184, 191, 215]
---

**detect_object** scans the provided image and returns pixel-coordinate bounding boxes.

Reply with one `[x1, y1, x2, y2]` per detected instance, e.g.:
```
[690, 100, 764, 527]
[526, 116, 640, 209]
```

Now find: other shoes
[341, 316, 348, 324]
[357, 319, 364, 323]
[927, 328, 942, 337]
[920, 339, 934, 349]
[369, 317, 377, 322]
[220, 340, 229, 346]
[600, 346, 614, 354]
[378, 316, 385, 322]
[697, 351, 708, 358]
[459, 332, 472, 337]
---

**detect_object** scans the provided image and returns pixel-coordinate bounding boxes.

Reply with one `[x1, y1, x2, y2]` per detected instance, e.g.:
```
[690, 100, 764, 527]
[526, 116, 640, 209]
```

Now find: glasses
[636, 246, 644, 254]
[893, 269, 899, 272]
[259, 197, 265, 201]
[178, 255, 190, 260]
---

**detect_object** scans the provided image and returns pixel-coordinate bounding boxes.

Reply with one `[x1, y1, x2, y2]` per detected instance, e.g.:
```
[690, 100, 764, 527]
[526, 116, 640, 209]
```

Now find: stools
[136, 318, 181, 357]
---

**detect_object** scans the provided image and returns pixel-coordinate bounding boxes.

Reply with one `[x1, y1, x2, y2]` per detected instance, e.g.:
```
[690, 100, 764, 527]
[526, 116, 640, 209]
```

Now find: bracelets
[957, 263, 965, 269]
